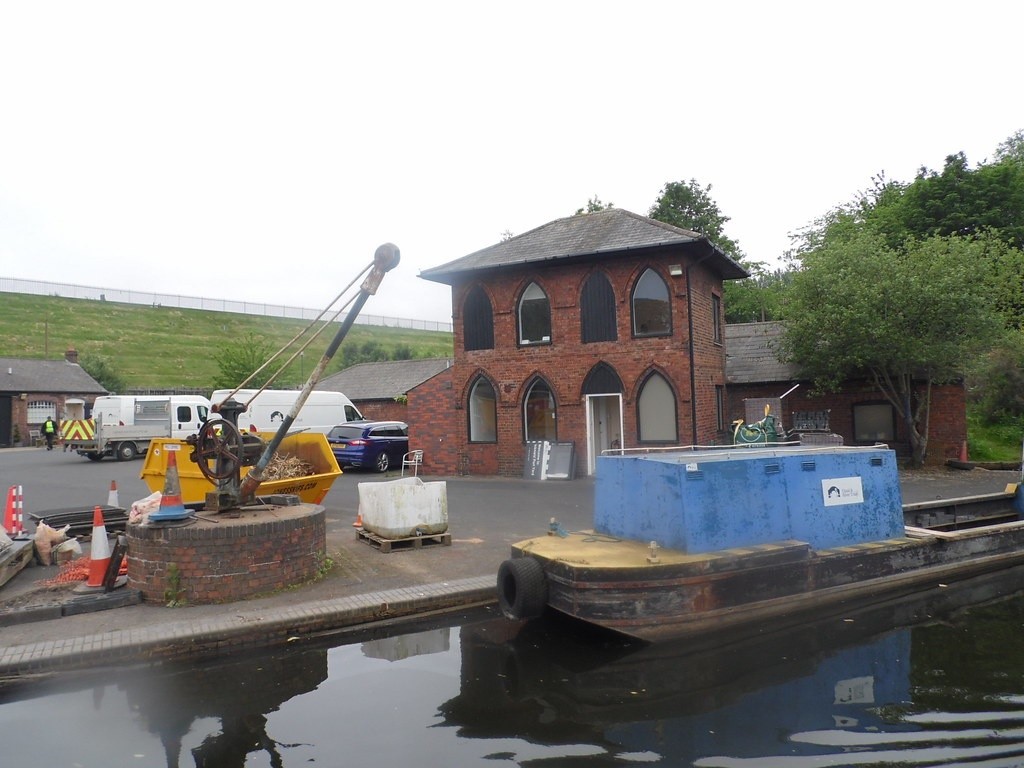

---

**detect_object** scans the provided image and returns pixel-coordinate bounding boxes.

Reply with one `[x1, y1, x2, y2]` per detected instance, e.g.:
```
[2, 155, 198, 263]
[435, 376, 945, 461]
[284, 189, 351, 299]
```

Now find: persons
[41, 416, 58, 451]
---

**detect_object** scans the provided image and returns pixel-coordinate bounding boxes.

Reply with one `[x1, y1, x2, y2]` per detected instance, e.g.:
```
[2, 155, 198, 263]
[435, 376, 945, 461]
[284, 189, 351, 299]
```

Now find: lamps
[669, 264, 682, 275]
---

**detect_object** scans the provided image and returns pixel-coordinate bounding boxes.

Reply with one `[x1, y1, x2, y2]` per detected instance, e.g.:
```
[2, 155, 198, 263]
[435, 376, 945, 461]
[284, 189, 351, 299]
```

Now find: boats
[497, 441, 1024, 646]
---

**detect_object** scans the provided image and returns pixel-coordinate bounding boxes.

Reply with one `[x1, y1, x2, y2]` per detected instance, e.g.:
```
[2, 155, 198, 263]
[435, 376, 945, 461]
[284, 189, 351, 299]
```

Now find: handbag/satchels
[129, 490, 162, 524]
[34, 519, 82, 566]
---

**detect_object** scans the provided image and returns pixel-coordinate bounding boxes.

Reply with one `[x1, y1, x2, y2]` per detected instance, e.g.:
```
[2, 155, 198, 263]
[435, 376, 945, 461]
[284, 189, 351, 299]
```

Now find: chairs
[402, 449, 423, 477]
[30, 430, 40, 446]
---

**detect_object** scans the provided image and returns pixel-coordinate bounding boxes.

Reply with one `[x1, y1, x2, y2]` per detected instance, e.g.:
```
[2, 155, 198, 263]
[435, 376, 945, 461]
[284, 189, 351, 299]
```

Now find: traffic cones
[147, 450, 197, 521]
[108, 479, 122, 510]
[3, 485, 15, 534]
[70, 505, 126, 593]
[352, 504, 363, 527]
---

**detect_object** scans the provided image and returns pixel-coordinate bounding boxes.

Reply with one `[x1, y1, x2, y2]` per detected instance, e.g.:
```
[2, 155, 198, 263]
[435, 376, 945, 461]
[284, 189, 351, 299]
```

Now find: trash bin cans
[142, 432, 342, 513]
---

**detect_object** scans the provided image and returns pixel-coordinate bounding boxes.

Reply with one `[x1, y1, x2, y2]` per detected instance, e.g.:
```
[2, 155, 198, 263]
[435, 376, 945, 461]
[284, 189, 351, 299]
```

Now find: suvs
[327, 420, 409, 474]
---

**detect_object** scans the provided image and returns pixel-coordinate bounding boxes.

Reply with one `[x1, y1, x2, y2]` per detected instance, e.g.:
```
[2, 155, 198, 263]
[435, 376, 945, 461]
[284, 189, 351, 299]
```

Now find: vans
[91, 394, 210, 455]
[208, 389, 368, 437]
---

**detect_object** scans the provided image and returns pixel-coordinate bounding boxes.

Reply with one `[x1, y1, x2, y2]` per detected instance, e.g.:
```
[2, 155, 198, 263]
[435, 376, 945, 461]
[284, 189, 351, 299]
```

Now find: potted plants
[13, 424, 22, 446]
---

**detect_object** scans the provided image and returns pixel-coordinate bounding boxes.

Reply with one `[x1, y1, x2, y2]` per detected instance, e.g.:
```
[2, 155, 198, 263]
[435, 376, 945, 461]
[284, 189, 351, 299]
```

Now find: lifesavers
[496, 556, 546, 620]
